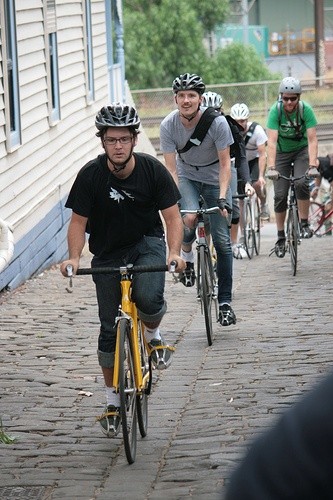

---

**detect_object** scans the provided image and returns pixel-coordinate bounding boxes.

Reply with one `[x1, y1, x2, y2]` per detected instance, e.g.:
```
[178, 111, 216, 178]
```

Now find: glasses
[103, 135, 134, 145]
[282, 96, 298, 101]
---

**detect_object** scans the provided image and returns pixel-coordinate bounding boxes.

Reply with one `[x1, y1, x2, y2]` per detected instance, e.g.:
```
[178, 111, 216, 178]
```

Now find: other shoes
[299, 219, 314, 237]
[231, 242, 243, 260]
[260, 202, 270, 220]
[272, 236, 286, 258]
[239, 235, 248, 249]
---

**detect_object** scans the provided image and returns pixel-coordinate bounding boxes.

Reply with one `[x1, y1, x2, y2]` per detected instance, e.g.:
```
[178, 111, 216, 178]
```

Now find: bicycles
[64, 258, 178, 465]
[234, 176, 262, 260]
[176, 199, 249, 347]
[303, 186, 333, 240]
[274, 171, 309, 276]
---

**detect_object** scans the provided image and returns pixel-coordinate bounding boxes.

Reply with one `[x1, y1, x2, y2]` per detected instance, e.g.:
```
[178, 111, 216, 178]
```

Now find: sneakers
[218, 302, 236, 326]
[178, 249, 196, 288]
[99, 402, 123, 438]
[147, 332, 175, 370]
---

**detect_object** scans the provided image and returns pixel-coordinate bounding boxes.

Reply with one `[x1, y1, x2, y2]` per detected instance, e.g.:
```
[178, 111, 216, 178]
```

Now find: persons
[64, 102, 186, 436]
[230, 102, 270, 219]
[267, 77, 319, 258]
[159, 73, 237, 326]
[201, 91, 256, 260]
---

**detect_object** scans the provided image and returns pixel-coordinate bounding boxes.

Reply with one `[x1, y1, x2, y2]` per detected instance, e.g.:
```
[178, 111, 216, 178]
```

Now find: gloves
[305, 165, 320, 180]
[267, 166, 281, 181]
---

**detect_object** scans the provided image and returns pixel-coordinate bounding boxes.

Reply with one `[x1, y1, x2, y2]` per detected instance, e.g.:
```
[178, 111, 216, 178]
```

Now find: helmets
[172, 72, 206, 95]
[200, 91, 223, 109]
[230, 103, 250, 121]
[95, 103, 141, 129]
[279, 77, 302, 94]
